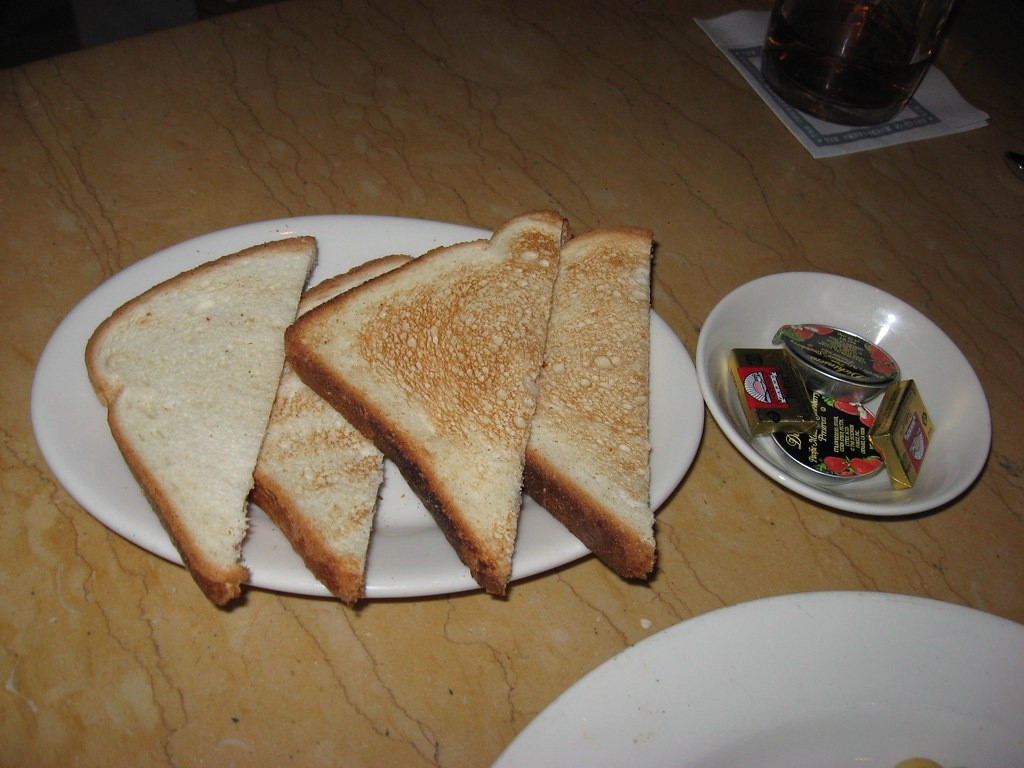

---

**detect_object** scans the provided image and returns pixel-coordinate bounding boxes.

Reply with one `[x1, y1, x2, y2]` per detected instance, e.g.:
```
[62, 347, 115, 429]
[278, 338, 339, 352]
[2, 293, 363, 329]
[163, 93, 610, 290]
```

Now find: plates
[490, 592, 1024, 768]
[696, 271, 993, 515]
[31, 214, 703, 598]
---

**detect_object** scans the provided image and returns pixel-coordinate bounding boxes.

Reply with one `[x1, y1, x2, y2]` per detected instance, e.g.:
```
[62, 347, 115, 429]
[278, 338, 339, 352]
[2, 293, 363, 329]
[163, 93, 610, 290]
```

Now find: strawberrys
[818, 396, 882, 477]
[864, 343, 897, 373]
[783, 324, 834, 341]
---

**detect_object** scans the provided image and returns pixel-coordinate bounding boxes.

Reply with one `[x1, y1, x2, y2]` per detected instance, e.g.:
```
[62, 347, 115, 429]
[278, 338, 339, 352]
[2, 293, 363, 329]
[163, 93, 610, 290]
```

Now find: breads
[248, 252, 414, 605]
[521, 222, 656, 581]
[85, 234, 317, 608]
[284, 208, 572, 597]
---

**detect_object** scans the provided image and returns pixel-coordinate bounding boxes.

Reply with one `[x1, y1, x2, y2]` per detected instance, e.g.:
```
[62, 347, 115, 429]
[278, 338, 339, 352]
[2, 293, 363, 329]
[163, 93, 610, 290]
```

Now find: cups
[763, 0, 952, 126]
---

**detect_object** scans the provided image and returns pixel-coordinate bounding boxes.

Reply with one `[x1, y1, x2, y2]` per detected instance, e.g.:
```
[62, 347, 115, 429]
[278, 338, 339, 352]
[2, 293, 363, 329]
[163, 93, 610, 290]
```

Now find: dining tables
[0, 0, 1024, 768]
